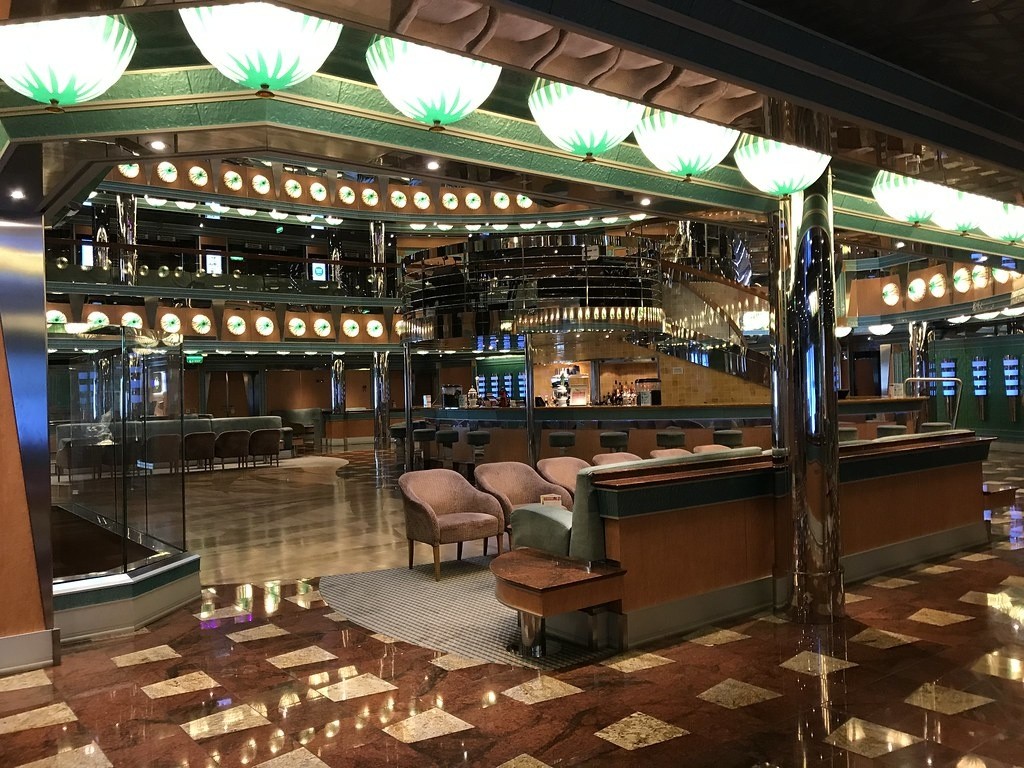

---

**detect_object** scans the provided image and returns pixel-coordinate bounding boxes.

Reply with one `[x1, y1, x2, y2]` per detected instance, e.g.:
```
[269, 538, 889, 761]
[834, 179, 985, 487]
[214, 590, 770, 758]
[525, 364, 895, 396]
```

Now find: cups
[442, 383, 477, 409]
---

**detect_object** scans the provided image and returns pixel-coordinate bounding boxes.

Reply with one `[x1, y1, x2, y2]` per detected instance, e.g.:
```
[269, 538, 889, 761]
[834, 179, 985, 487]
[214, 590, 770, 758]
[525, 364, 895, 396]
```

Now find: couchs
[271, 409, 323, 437]
[511, 446, 762, 559]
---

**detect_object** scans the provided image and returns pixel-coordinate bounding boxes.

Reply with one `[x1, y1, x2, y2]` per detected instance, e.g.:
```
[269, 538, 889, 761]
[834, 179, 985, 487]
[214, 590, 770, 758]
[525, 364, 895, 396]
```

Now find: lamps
[0, 3, 1024, 245]
[882, 265, 1022, 306]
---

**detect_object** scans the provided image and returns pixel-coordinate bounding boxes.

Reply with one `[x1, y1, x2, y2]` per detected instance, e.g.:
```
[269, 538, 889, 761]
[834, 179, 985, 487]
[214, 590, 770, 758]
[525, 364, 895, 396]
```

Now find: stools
[390, 420, 490, 470]
[548, 431, 743, 450]
[838, 422, 952, 442]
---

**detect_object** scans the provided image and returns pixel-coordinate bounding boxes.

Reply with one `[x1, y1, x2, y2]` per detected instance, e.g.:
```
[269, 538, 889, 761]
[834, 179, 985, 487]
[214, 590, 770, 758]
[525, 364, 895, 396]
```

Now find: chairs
[399, 469, 505, 582]
[99, 436, 141, 478]
[474, 462, 573, 556]
[215, 430, 250, 468]
[250, 429, 280, 467]
[693, 444, 731, 455]
[180, 432, 216, 473]
[56, 440, 100, 482]
[136, 434, 180, 476]
[537, 457, 591, 504]
[650, 448, 693, 459]
[592, 452, 642, 466]
